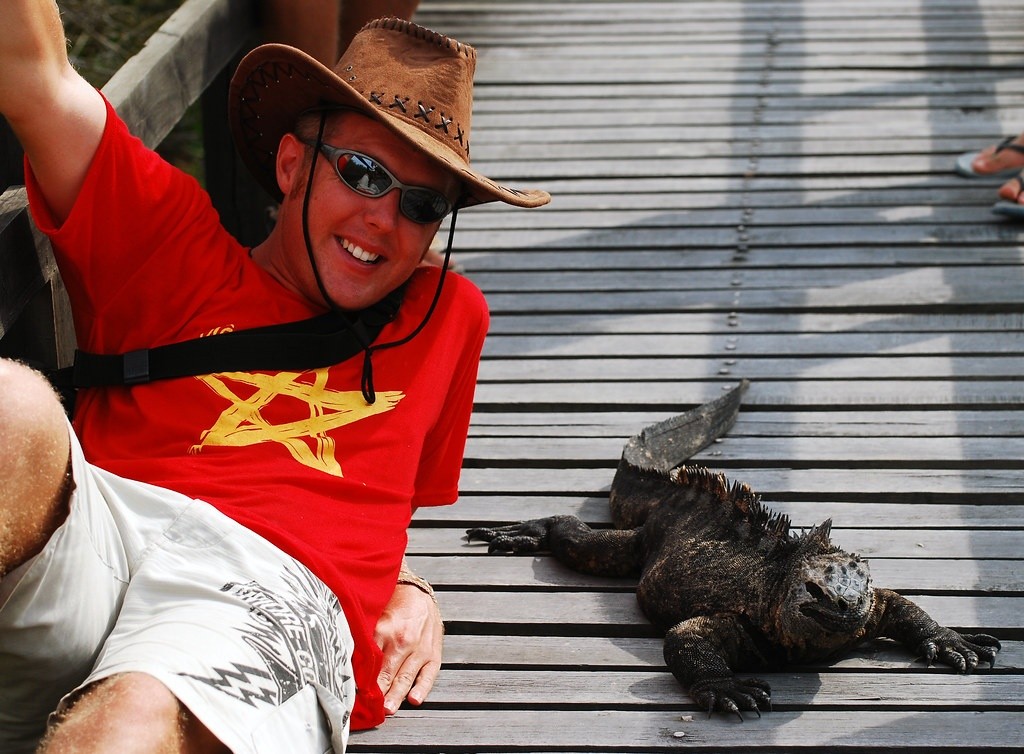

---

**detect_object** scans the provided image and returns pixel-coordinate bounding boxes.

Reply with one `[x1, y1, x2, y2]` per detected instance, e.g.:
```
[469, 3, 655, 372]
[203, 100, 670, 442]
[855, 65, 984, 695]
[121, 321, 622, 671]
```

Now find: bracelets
[393, 570, 437, 599]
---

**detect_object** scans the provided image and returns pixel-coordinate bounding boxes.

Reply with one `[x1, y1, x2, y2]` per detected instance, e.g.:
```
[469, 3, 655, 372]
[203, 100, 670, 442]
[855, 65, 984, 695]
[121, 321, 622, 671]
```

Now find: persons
[0, 0, 550, 754]
[954, 129, 1024, 215]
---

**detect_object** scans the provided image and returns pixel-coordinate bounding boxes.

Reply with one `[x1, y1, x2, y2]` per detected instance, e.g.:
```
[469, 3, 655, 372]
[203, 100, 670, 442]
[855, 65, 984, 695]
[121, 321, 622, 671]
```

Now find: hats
[226, 19, 552, 213]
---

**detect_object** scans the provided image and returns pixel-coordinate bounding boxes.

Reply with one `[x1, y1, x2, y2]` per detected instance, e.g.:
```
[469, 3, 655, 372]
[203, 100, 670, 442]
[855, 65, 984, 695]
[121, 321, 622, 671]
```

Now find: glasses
[300, 138, 453, 224]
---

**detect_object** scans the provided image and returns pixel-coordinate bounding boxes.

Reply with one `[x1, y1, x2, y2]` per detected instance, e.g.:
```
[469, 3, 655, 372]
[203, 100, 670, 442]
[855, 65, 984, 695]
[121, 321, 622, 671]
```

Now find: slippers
[992, 173, 1024, 214]
[955, 137, 1024, 180]
